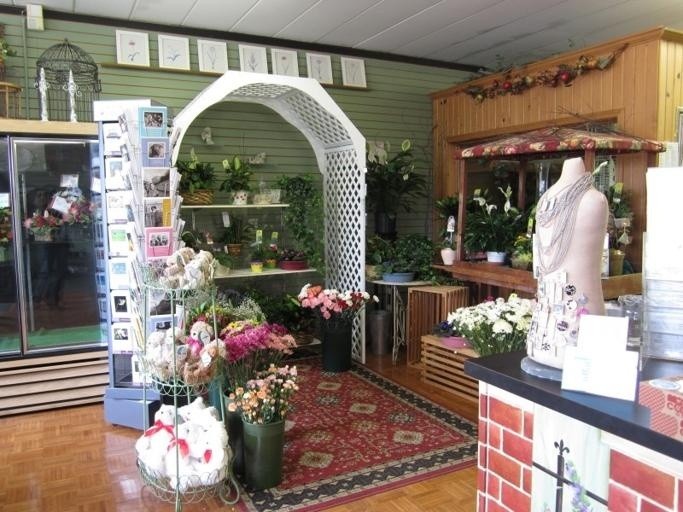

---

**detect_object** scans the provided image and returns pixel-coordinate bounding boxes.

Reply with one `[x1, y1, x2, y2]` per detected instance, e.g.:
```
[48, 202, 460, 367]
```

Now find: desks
[370, 281, 432, 367]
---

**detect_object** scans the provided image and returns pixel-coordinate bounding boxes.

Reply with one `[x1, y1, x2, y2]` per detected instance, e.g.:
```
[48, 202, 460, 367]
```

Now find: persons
[150, 205, 162, 227]
[147, 183, 160, 197]
[526, 157, 609, 370]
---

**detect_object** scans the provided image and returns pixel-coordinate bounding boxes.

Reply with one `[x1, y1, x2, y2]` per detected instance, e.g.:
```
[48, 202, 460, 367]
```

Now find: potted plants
[359, 232, 434, 282]
[155, 149, 308, 274]
[605, 183, 636, 276]
[428, 194, 457, 266]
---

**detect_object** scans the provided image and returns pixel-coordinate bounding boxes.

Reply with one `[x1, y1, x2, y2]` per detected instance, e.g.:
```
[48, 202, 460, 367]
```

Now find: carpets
[217, 354, 480, 506]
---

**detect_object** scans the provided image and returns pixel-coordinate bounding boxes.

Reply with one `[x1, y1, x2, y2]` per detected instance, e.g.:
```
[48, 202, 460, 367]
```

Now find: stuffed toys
[135, 397, 230, 492]
[145, 317, 225, 385]
[159, 246, 214, 290]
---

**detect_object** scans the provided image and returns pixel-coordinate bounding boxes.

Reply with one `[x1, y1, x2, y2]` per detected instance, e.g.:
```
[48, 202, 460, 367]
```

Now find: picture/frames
[89, 105, 176, 375]
[112, 29, 370, 88]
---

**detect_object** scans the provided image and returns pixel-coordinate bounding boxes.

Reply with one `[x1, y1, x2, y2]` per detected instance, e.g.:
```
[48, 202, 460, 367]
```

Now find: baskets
[137, 458, 227, 504]
[132, 351, 217, 395]
[142, 261, 211, 300]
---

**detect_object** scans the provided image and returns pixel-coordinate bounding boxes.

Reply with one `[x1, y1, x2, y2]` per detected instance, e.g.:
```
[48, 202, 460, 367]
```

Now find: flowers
[21, 199, 92, 233]
[188, 298, 303, 422]
[365, 138, 433, 213]
[465, 183, 528, 252]
[440, 293, 537, 358]
[291, 281, 368, 321]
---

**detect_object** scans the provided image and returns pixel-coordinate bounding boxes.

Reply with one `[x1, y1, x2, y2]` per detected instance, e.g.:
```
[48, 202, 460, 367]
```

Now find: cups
[618, 294, 641, 347]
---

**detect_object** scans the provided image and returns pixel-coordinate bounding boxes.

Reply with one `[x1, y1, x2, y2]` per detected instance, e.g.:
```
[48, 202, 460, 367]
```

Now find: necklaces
[535, 171, 595, 275]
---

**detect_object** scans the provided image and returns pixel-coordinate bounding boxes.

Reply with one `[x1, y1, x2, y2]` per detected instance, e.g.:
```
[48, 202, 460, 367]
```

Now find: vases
[315, 320, 354, 373]
[25, 223, 91, 242]
[484, 250, 508, 263]
[375, 211, 399, 237]
[204, 366, 292, 489]
